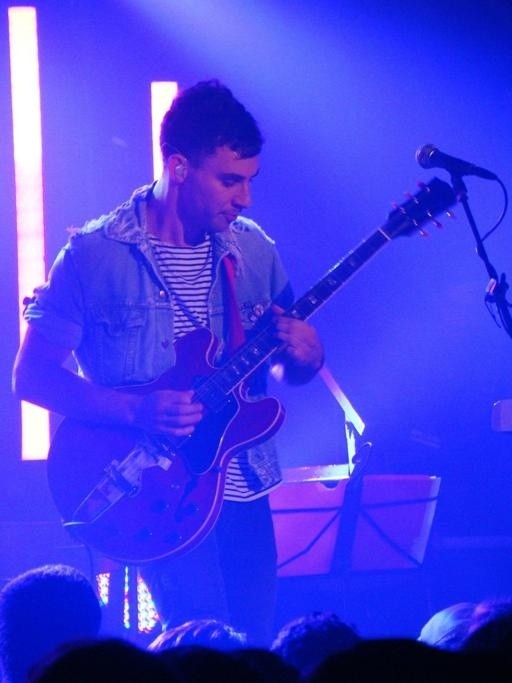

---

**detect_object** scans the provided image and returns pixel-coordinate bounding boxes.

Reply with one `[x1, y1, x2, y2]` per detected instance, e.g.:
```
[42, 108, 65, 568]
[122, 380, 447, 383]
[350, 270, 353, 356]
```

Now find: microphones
[415, 143, 498, 183]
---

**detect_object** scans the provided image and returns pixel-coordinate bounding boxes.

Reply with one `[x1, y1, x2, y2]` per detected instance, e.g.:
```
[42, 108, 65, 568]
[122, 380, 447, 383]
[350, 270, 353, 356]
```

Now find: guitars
[47, 175, 460, 563]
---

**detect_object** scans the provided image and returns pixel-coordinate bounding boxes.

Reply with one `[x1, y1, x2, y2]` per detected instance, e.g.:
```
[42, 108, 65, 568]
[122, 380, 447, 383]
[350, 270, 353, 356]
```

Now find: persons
[11, 77, 325, 629]
